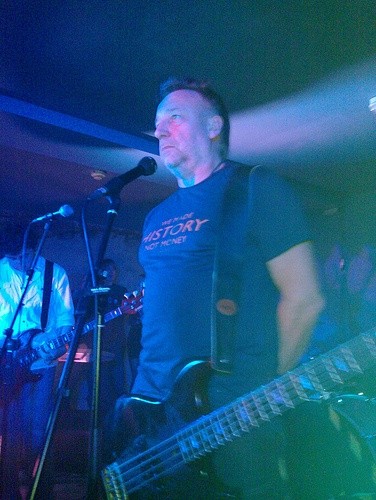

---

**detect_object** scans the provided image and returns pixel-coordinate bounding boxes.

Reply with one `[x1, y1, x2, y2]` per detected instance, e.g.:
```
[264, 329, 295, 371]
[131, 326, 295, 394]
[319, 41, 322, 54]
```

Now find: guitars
[0, 289, 145, 403]
[102, 324, 375, 499]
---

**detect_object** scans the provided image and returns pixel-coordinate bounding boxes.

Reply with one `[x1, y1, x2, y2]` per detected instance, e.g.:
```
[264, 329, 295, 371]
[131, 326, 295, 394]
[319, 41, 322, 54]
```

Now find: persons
[126, 75, 327, 500]
[0, 217, 76, 500]
[79, 258, 141, 397]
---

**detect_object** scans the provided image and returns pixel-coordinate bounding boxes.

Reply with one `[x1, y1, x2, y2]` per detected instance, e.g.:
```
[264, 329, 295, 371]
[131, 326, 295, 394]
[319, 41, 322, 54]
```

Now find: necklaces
[212, 159, 226, 174]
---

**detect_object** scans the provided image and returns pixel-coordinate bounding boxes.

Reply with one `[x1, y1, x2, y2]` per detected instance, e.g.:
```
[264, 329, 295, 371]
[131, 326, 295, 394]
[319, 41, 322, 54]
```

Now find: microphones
[33, 204, 74, 223]
[88, 157, 157, 202]
